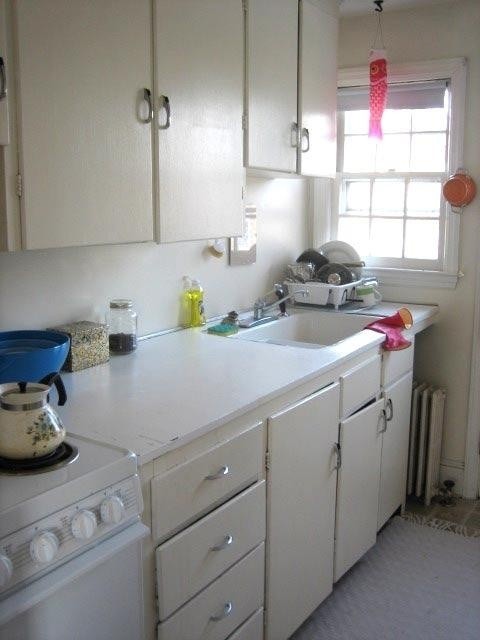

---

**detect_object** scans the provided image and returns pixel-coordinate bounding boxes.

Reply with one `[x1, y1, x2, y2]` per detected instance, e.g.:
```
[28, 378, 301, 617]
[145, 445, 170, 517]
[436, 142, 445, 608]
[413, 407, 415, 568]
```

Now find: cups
[355, 285, 382, 308]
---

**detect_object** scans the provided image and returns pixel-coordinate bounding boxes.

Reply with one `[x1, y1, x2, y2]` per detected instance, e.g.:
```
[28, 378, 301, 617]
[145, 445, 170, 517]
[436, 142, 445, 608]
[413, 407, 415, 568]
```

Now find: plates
[314, 240, 360, 263]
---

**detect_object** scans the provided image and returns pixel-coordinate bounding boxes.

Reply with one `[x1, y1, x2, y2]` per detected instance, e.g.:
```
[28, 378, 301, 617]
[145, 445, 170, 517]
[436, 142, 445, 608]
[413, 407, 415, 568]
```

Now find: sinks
[201, 306, 389, 348]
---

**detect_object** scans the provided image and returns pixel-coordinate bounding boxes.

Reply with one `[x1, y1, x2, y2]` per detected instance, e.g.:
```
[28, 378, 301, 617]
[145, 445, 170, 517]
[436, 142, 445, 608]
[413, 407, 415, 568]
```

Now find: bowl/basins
[0, 330, 70, 383]
[315, 264, 352, 285]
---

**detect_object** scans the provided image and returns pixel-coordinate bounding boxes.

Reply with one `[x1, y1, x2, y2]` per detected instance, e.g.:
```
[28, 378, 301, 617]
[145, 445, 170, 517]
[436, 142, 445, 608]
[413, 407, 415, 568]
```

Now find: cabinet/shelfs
[246, 1, 341, 178]
[140, 330, 412, 640]
[1, 1, 247, 253]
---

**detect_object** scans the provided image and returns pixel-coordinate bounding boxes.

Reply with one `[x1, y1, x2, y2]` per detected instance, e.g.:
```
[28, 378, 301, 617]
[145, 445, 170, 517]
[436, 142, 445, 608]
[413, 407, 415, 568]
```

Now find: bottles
[106, 299, 137, 354]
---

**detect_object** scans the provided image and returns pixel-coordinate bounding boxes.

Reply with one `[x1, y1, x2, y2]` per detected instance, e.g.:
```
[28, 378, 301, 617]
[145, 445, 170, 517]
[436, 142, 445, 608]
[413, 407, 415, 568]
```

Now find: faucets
[252, 289, 310, 319]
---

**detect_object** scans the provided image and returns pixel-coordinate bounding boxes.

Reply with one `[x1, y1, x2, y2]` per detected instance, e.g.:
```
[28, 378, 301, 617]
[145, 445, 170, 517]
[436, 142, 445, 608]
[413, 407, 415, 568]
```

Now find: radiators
[407, 379, 452, 507]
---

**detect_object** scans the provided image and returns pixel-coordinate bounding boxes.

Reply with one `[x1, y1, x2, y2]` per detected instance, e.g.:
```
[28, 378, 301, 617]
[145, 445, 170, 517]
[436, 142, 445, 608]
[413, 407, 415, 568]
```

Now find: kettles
[0, 372, 67, 460]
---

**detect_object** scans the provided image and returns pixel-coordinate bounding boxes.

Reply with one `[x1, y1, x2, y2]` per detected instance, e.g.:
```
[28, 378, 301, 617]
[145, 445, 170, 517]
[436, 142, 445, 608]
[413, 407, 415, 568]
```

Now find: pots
[443, 167, 476, 213]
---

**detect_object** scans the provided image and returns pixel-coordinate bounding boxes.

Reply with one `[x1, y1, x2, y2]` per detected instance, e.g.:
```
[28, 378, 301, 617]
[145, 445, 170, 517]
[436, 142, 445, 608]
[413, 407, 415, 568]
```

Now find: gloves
[364, 307, 414, 351]
